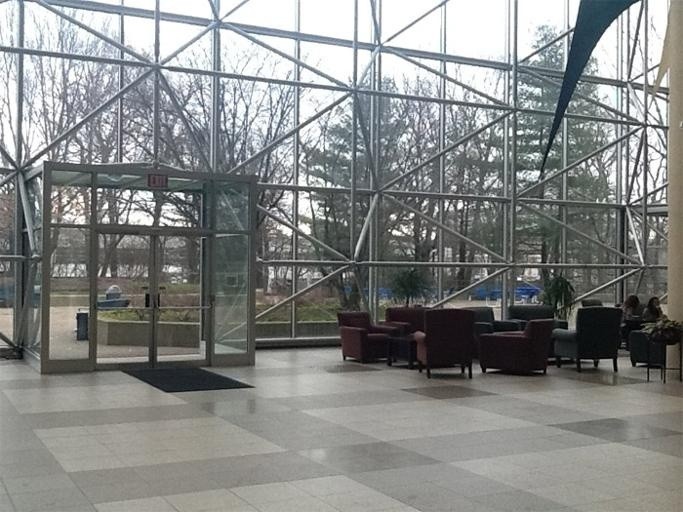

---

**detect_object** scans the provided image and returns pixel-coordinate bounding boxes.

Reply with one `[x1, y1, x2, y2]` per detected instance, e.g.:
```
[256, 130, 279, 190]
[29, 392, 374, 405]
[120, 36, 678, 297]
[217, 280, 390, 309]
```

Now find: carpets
[120, 366, 256, 393]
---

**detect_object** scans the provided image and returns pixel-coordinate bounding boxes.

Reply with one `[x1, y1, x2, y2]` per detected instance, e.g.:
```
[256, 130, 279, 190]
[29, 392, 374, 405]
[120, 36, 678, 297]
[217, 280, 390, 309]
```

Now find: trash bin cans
[106, 285, 121, 300]
[77, 313, 88, 340]
[145, 293, 160, 307]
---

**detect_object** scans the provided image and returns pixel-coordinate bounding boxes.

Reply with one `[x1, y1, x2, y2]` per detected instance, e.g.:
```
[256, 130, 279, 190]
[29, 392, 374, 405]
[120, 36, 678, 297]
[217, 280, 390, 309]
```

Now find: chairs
[628, 327, 682, 385]
[337, 299, 623, 383]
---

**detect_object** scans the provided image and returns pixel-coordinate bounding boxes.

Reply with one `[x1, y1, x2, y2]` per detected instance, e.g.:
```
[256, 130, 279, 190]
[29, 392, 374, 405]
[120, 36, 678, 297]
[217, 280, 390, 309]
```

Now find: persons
[641, 297, 662, 319]
[619, 296, 641, 330]
